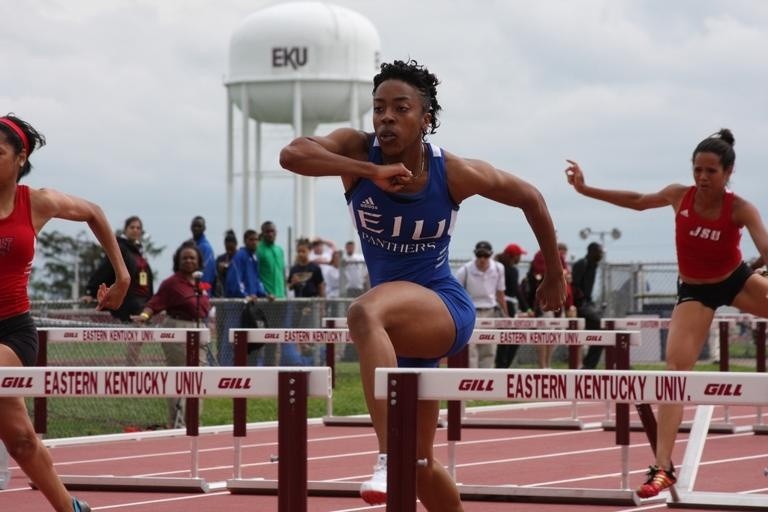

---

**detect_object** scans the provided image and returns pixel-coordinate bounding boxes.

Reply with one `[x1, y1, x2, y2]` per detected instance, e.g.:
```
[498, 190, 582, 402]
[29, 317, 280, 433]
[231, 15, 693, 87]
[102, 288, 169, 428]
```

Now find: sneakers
[360, 454, 388, 505]
[636, 460, 676, 498]
[73, 496, 90, 512]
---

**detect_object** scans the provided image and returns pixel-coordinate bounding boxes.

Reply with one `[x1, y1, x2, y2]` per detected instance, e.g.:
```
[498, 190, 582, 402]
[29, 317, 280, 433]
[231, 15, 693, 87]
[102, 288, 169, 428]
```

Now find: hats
[505, 244, 527, 256]
[475, 241, 491, 255]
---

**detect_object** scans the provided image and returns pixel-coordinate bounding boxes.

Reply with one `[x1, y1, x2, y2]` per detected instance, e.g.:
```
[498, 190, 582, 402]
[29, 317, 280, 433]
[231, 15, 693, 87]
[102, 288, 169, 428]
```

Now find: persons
[280, 59, 568, 512]
[565, 128, 768, 498]
[455, 243, 603, 370]
[0, 114, 131, 512]
[131, 239, 212, 429]
[85, 218, 365, 364]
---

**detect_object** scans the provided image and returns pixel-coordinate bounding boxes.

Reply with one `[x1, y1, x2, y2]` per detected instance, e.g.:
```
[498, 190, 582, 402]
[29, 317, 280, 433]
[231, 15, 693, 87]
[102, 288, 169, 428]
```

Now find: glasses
[477, 253, 489, 257]
